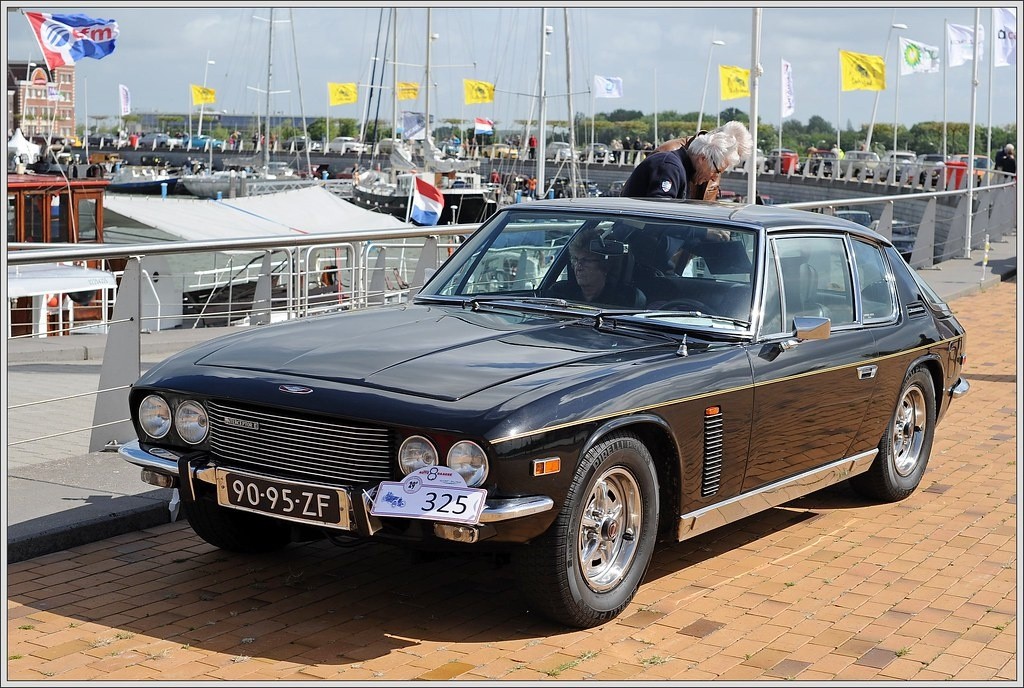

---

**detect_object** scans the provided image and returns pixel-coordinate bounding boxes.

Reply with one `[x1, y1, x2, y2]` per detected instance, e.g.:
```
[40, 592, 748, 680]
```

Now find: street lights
[198, 61, 216, 140]
[697, 39, 726, 133]
[524, 51, 553, 151]
[20, 63, 37, 130]
[864, 22, 908, 151]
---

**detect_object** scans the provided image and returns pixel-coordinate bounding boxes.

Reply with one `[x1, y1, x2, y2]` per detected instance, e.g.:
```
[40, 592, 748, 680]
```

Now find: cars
[839, 152, 881, 180]
[80, 128, 404, 154]
[579, 142, 615, 164]
[544, 142, 578, 162]
[951, 155, 996, 185]
[913, 154, 951, 187]
[875, 151, 918, 182]
[481, 142, 519, 161]
[502, 171, 916, 263]
[116, 198, 968, 631]
[763, 149, 799, 173]
[798, 150, 841, 177]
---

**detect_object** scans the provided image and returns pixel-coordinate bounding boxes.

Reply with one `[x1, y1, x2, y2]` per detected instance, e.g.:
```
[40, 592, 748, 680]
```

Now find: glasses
[569, 255, 598, 266]
[710, 155, 721, 176]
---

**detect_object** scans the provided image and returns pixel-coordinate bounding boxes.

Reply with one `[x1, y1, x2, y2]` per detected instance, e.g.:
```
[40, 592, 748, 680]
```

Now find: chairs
[634, 264, 665, 300]
[750, 256, 831, 335]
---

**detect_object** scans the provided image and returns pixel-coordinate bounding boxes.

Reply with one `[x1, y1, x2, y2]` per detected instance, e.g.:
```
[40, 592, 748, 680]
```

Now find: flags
[992, 8, 1016, 68]
[719, 65, 751, 101]
[781, 58, 796, 119]
[474, 117, 494, 135]
[328, 82, 358, 106]
[463, 79, 496, 105]
[947, 24, 985, 68]
[402, 111, 435, 140]
[898, 37, 940, 76]
[23, 11, 120, 71]
[839, 50, 887, 92]
[594, 75, 623, 98]
[119, 83, 131, 117]
[397, 81, 420, 100]
[191, 85, 217, 106]
[410, 176, 444, 226]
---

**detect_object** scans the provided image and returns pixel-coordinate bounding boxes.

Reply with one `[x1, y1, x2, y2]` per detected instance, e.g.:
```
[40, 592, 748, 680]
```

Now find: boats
[106, 166, 179, 195]
[6, 164, 478, 340]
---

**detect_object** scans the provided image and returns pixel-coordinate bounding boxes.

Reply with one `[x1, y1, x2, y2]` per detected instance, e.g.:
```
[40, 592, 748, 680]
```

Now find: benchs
[666, 277, 885, 321]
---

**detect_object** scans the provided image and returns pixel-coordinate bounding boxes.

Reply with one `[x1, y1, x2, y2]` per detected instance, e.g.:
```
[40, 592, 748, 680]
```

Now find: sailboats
[178, 7, 603, 226]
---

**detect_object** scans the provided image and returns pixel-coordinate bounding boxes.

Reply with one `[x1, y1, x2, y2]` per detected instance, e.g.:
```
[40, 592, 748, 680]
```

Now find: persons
[611, 137, 653, 164]
[610, 132, 740, 276]
[807, 143, 817, 157]
[440, 134, 478, 160]
[490, 169, 501, 183]
[529, 134, 538, 158]
[994, 143, 1016, 181]
[653, 121, 753, 270]
[830, 143, 841, 158]
[527, 175, 537, 199]
[542, 229, 627, 306]
